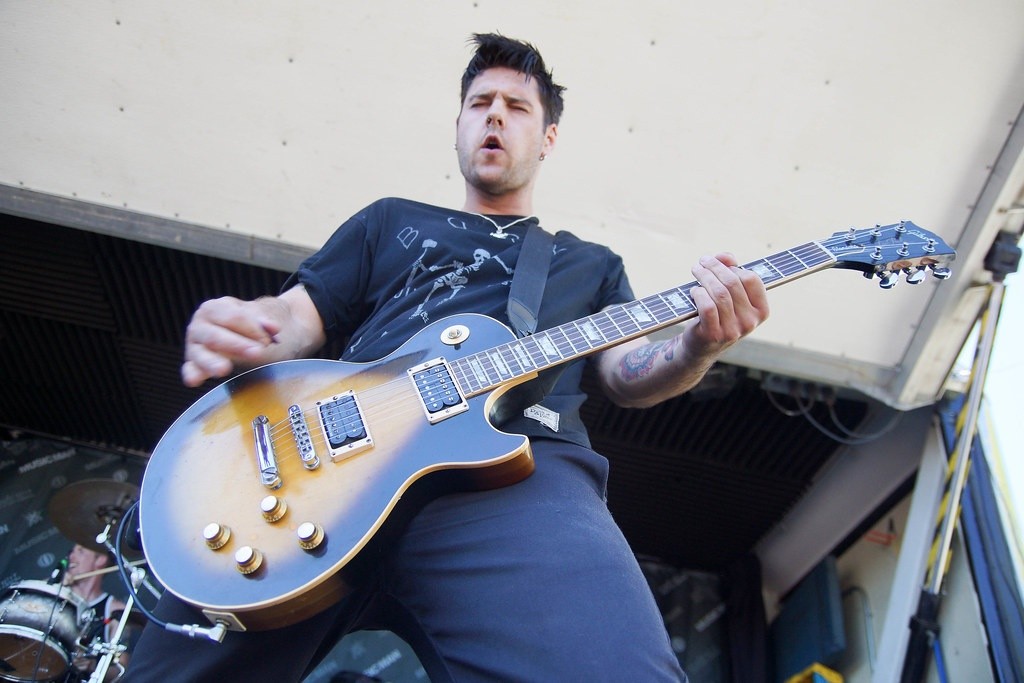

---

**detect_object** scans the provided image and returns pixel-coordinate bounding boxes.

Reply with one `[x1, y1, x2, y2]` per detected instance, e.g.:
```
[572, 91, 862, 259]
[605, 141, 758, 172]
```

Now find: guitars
[133, 212, 960, 639]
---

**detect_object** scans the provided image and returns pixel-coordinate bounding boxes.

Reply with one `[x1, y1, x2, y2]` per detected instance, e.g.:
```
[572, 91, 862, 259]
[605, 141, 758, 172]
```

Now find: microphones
[47, 558, 66, 584]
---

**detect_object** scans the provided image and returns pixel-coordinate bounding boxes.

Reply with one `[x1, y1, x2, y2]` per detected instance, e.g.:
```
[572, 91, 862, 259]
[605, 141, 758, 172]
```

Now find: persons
[46, 542, 129, 665]
[110, 30, 772, 683]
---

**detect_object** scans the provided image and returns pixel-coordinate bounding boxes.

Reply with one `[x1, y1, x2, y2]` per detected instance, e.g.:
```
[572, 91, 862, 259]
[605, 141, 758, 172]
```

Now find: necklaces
[469, 212, 538, 239]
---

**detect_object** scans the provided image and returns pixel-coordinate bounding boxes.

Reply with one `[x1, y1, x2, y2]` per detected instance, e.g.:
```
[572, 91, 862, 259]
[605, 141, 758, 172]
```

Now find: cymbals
[43, 478, 144, 558]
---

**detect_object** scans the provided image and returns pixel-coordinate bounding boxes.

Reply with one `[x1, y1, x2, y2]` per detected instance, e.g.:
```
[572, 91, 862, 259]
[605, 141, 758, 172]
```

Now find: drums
[1, 578, 93, 683]
